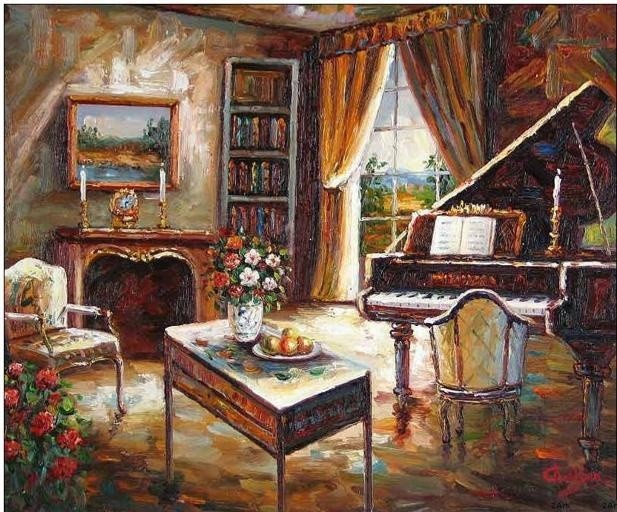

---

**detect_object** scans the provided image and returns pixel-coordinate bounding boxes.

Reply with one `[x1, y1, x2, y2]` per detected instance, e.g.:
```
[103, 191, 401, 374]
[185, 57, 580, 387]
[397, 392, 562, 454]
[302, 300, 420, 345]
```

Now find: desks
[162, 317, 372, 512]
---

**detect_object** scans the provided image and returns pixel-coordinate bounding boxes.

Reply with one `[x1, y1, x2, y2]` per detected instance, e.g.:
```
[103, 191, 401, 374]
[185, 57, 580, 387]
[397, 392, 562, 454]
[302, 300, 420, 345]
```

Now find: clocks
[106, 189, 139, 229]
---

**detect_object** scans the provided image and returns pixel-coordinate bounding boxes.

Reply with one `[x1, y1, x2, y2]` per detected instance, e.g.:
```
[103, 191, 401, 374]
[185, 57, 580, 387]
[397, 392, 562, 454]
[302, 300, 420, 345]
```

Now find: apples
[262, 328, 314, 357]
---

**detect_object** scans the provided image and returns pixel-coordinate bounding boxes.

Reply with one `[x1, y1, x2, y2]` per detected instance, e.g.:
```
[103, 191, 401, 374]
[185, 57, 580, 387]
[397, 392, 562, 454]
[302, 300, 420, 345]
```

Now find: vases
[224, 299, 261, 342]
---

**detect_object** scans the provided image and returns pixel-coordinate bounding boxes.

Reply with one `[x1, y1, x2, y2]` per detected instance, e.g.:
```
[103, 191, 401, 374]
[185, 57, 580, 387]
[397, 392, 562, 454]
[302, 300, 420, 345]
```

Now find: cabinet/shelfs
[218, 55, 299, 301]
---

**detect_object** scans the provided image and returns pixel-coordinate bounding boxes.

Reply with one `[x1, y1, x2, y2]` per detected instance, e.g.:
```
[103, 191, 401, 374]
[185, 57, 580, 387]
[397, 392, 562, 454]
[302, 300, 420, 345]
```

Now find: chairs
[427, 290, 526, 446]
[4, 259, 128, 415]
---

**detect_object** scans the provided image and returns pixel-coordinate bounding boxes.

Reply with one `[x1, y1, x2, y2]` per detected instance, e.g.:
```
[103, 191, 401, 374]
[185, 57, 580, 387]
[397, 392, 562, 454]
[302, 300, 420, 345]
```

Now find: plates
[251, 341, 322, 363]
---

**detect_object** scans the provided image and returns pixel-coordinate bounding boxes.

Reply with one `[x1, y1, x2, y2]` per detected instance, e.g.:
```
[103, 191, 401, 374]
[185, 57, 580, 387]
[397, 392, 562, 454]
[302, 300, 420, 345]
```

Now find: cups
[196, 337, 208, 347]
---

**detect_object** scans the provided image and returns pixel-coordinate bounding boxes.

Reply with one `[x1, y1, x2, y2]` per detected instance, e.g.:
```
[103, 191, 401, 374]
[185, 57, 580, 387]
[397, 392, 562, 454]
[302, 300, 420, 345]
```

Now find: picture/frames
[64, 95, 180, 188]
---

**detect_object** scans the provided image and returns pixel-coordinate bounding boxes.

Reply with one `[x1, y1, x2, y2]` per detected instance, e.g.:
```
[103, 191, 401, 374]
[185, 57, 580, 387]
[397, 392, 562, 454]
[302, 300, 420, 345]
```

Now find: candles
[80, 164, 87, 201]
[553, 169, 562, 207]
[159, 163, 167, 201]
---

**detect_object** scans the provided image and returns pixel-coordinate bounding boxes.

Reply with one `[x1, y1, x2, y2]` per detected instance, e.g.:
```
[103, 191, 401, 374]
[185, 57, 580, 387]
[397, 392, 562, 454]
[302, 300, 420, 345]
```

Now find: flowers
[4, 360, 94, 512]
[197, 229, 284, 305]
